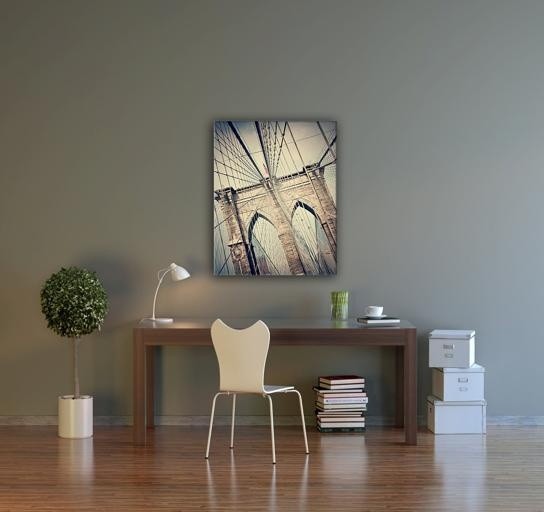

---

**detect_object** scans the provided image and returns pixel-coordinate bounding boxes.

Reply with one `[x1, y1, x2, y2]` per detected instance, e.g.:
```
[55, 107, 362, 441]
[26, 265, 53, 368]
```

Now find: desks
[134, 317, 416, 444]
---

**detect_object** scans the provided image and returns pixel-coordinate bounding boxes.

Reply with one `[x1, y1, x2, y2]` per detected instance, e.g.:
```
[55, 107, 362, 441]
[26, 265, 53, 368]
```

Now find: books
[313, 375, 369, 433]
[357, 315, 401, 325]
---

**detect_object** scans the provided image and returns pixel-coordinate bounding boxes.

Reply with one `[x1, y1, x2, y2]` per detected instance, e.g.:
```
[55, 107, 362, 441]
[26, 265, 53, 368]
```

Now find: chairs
[205, 318, 310, 464]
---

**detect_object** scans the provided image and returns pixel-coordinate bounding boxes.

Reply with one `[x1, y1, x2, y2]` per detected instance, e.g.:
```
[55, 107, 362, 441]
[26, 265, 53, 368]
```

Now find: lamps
[151, 263, 190, 322]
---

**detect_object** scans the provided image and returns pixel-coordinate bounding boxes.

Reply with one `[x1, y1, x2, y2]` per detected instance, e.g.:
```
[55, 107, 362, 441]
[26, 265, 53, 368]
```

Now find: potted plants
[40, 266, 110, 440]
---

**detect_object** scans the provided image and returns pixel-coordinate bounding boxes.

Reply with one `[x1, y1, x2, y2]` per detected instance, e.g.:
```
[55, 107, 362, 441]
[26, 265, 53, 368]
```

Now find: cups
[365, 305, 384, 315]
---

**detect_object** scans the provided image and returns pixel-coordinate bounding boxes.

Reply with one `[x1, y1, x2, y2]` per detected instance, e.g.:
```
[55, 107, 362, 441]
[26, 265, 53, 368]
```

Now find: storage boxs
[429, 329, 476, 368]
[432, 364, 484, 401]
[426, 395, 487, 435]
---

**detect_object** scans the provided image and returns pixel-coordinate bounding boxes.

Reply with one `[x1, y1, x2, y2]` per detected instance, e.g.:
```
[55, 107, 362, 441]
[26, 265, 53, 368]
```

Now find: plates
[364, 314, 388, 319]
